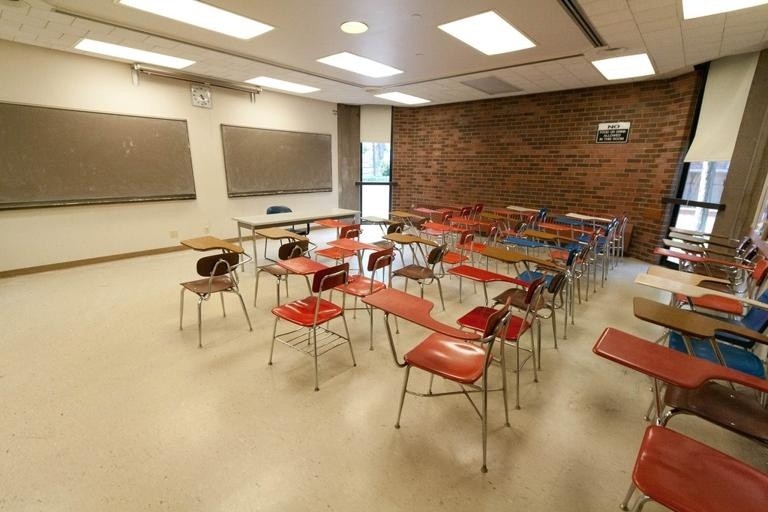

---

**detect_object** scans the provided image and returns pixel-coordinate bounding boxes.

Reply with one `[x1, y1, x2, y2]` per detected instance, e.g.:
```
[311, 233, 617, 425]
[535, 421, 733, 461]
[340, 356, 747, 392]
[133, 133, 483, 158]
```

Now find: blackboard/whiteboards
[2, 102, 196, 211]
[219, 124, 332, 199]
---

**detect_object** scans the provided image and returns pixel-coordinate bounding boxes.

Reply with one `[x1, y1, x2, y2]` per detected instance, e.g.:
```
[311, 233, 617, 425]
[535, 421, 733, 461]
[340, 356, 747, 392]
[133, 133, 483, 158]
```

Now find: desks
[525, 229, 590, 316]
[254, 229, 313, 318]
[361, 287, 513, 473]
[321, 237, 399, 350]
[504, 237, 578, 326]
[448, 265, 547, 410]
[633, 296, 768, 445]
[633, 227, 768, 342]
[231, 209, 361, 275]
[538, 222, 601, 301]
[383, 232, 448, 312]
[555, 215, 614, 293]
[565, 212, 619, 288]
[268, 257, 356, 391]
[391, 197, 548, 239]
[360, 215, 406, 274]
[480, 246, 567, 372]
[575, 208, 628, 271]
[422, 223, 478, 303]
[592, 326, 768, 512]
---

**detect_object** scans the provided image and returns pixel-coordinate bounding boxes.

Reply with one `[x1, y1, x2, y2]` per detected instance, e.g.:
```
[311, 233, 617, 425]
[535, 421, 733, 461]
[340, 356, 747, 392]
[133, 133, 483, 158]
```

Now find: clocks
[190, 84, 213, 109]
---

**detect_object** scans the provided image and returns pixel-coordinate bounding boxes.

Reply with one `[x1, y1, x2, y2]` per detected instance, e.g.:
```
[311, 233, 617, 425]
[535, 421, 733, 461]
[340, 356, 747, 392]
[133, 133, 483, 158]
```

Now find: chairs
[177, 235, 253, 346]
[264, 206, 310, 263]
